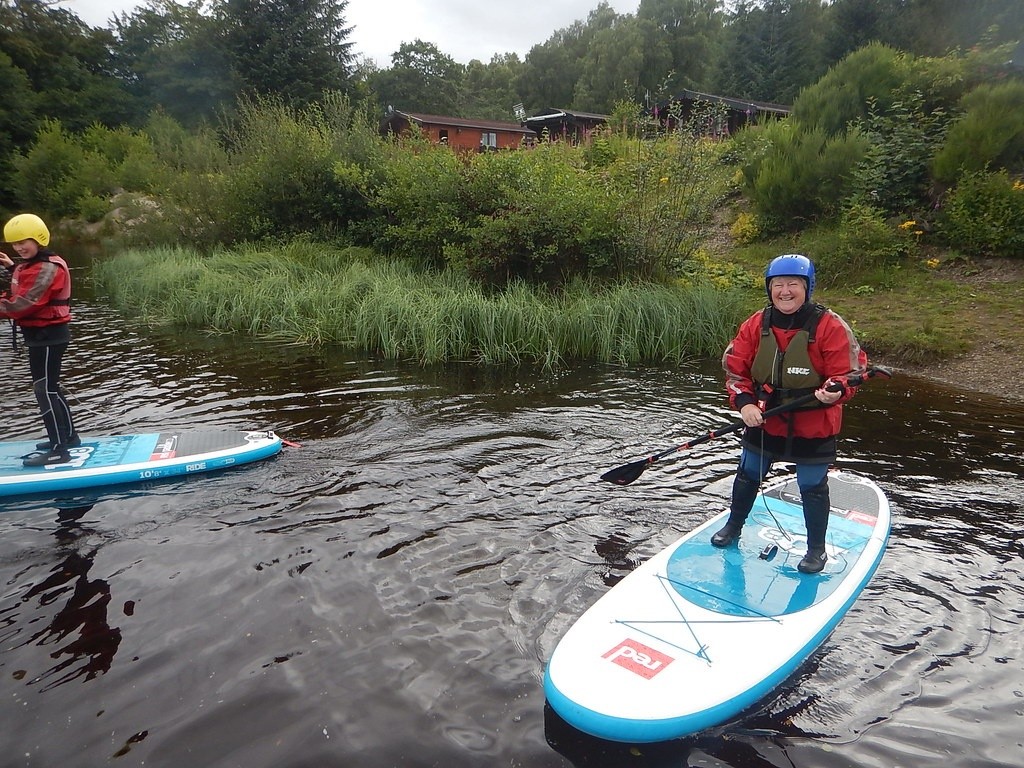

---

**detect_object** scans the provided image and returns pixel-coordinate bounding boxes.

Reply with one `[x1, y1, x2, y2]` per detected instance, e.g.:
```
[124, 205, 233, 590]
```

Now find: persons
[711, 255, 866, 574]
[0, 214, 80, 467]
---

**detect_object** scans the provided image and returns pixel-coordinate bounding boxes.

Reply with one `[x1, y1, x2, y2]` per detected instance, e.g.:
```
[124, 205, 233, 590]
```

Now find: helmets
[766, 254, 816, 302]
[3, 214, 50, 247]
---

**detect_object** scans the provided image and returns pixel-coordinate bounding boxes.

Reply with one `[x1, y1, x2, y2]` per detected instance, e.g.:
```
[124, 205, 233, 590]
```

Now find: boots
[798, 476, 830, 573]
[711, 469, 760, 547]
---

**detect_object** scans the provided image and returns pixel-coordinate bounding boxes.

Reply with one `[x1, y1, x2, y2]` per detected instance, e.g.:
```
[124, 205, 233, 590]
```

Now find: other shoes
[23, 450, 72, 466]
[36, 433, 81, 450]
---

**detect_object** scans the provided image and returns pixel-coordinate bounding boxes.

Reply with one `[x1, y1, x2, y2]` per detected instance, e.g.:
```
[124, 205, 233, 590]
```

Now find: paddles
[596, 364, 895, 488]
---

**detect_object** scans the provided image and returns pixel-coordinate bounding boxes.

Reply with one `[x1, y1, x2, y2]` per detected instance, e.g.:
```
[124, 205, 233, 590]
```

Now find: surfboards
[0, 426, 282, 500]
[543, 469, 893, 744]
